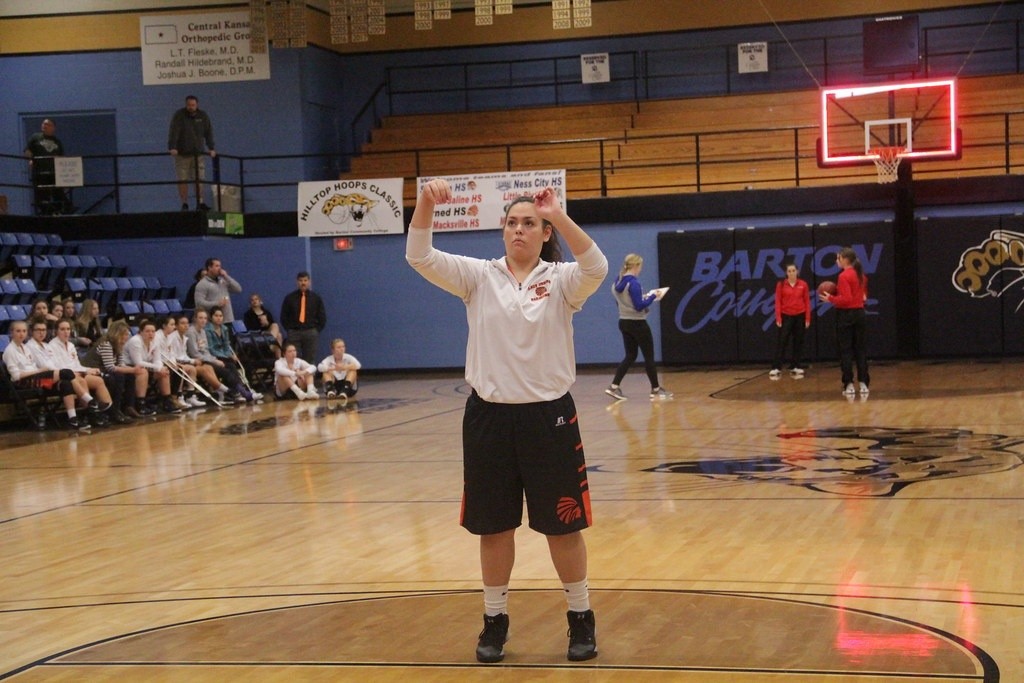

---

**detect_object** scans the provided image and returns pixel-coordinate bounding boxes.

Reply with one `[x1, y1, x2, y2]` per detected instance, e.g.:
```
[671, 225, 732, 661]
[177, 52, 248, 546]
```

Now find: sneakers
[606, 400, 627, 413]
[65, 390, 263, 429]
[650, 386, 674, 397]
[605, 385, 625, 400]
[650, 397, 673, 405]
[476, 613, 512, 663]
[566, 608, 599, 661]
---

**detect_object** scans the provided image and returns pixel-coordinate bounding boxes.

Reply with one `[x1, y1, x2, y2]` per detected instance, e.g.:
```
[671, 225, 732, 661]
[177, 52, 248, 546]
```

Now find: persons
[280, 271, 326, 393]
[48, 280, 85, 301]
[168, 94, 218, 212]
[768, 262, 812, 376]
[604, 253, 674, 400]
[2, 299, 118, 430]
[84, 322, 146, 423]
[0, 195, 11, 216]
[402, 178, 607, 661]
[185, 258, 242, 356]
[24, 118, 80, 216]
[272, 344, 319, 401]
[816, 247, 869, 394]
[244, 294, 283, 360]
[121, 304, 265, 416]
[317, 338, 361, 399]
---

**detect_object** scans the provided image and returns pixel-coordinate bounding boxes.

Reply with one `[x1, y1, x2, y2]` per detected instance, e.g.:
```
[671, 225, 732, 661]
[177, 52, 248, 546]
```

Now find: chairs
[0, 230, 282, 428]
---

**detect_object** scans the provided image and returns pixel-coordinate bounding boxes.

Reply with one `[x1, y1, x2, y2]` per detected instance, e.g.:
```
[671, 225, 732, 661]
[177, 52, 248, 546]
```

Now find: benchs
[340, 73, 1023, 205]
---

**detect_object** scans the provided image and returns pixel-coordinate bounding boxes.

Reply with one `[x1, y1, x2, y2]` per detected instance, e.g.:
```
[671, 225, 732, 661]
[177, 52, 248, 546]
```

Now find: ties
[300, 292, 306, 323]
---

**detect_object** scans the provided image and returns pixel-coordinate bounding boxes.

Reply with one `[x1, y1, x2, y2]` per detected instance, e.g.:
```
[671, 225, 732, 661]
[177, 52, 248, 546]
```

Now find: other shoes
[306, 390, 319, 399]
[769, 368, 780, 375]
[327, 388, 337, 399]
[842, 383, 855, 394]
[860, 382, 868, 393]
[298, 393, 308, 401]
[339, 391, 347, 399]
[791, 368, 804, 373]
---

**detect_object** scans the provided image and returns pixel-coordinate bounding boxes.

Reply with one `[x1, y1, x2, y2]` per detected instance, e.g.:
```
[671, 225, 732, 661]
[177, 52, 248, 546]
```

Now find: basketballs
[817, 281, 837, 296]
